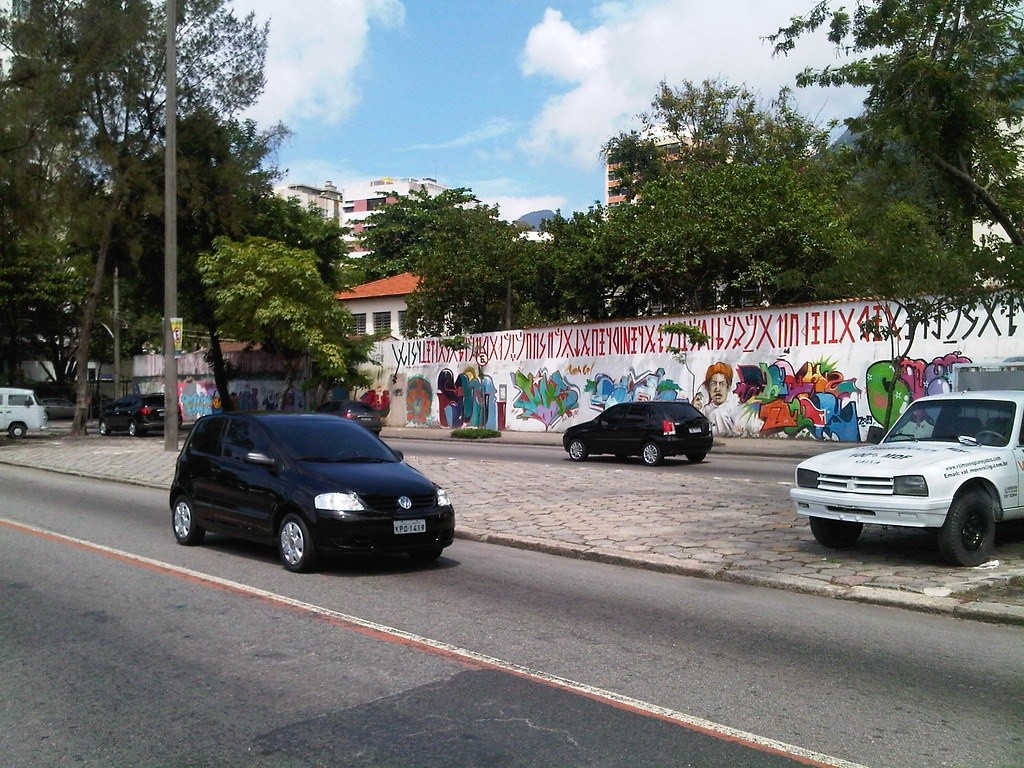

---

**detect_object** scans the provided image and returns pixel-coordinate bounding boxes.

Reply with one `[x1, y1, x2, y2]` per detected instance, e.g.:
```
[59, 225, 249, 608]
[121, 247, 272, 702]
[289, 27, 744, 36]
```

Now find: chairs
[958, 416, 983, 434]
[986, 417, 1011, 434]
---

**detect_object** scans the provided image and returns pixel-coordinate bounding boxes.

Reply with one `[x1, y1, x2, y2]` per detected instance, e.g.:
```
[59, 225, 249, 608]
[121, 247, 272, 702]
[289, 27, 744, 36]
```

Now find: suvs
[99, 393, 183, 436]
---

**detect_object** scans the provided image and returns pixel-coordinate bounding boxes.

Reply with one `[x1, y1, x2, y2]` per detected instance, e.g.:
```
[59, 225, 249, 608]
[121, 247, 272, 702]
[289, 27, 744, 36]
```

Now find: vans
[0, 388, 49, 438]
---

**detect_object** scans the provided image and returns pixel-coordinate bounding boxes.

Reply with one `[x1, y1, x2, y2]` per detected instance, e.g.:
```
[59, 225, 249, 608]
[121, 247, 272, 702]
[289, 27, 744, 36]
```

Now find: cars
[562, 402, 713, 465]
[313, 402, 383, 438]
[168, 409, 455, 575]
[40, 397, 77, 419]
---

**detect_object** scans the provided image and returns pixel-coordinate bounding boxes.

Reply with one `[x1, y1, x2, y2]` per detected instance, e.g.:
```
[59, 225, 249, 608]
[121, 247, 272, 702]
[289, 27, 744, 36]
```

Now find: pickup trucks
[789, 360, 1023, 568]
